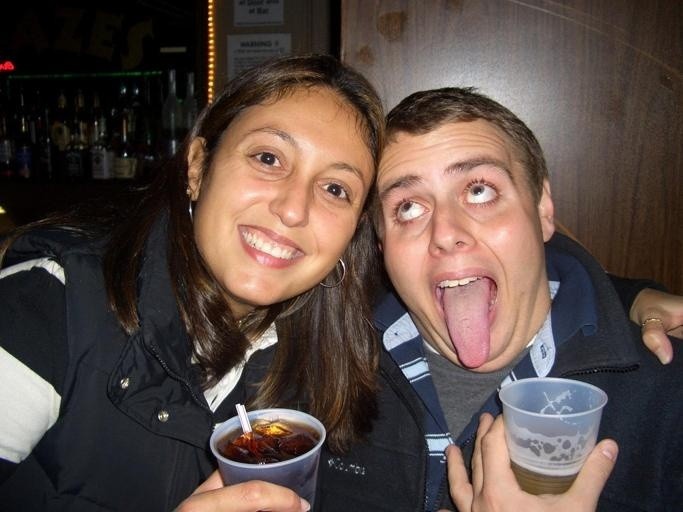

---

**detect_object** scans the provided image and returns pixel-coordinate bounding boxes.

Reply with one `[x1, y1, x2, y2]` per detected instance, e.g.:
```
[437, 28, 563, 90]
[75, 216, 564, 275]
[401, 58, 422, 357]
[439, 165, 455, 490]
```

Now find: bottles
[0, 69, 201, 184]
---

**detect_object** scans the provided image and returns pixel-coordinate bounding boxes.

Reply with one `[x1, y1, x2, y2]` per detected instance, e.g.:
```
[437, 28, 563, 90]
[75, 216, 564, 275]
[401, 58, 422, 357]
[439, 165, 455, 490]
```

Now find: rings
[640, 318, 663, 329]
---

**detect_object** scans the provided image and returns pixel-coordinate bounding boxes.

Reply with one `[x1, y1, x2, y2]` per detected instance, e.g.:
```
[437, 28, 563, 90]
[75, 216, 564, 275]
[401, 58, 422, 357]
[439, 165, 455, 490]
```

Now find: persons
[331, 86, 682, 511]
[0, 49, 683, 512]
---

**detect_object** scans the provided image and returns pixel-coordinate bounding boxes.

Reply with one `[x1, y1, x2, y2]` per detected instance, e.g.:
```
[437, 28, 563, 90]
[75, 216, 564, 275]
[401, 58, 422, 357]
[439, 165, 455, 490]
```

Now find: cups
[210, 408, 326, 512]
[499, 377, 608, 496]
